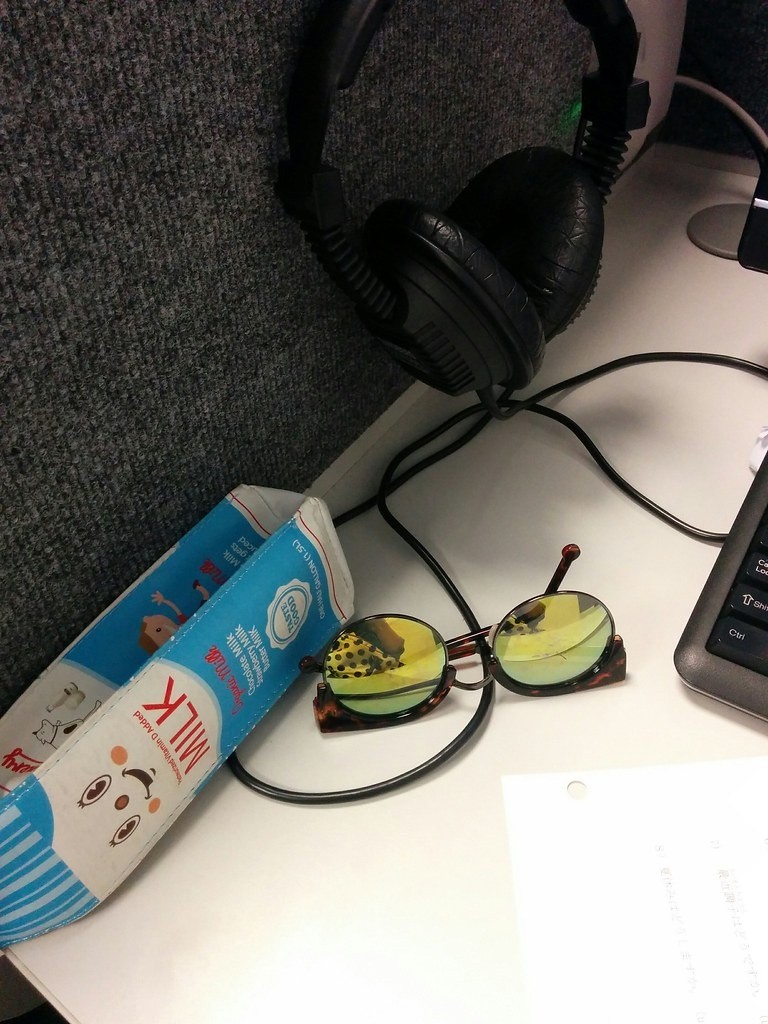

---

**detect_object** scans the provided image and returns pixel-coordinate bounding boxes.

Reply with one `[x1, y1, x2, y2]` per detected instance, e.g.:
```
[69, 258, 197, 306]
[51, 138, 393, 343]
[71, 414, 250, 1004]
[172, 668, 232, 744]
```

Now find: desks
[0, 133, 767, 1023]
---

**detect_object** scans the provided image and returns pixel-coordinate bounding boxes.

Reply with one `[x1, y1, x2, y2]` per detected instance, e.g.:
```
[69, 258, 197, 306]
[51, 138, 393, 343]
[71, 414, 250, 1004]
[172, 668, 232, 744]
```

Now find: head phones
[275, 3, 650, 391]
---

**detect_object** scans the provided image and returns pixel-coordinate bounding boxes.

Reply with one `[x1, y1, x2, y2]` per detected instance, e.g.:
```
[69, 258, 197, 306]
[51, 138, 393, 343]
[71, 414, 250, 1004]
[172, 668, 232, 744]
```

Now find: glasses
[299, 541, 627, 734]
